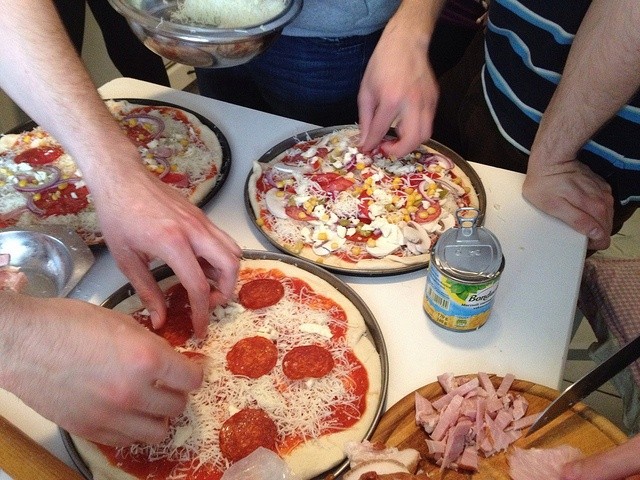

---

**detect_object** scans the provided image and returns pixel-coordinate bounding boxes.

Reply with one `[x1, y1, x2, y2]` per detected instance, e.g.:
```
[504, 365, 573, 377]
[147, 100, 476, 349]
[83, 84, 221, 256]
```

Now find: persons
[564, 432, 640, 480]
[0, 0, 246, 448]
[461, 0, 640, 251]
[214, 0, 450, 159]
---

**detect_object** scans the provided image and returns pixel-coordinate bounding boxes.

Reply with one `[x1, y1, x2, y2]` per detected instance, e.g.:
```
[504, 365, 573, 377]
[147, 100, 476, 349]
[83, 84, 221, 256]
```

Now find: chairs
[570, 258, 640, 438]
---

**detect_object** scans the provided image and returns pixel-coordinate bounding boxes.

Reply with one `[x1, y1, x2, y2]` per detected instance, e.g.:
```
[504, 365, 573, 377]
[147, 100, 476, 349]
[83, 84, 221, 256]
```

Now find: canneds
[423, 208, 505, 333]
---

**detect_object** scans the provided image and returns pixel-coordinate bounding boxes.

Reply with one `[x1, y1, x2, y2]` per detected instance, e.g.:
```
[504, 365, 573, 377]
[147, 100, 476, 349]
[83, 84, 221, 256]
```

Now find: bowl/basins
[0, 225, 96, 298]
[109, 0, 304, 69]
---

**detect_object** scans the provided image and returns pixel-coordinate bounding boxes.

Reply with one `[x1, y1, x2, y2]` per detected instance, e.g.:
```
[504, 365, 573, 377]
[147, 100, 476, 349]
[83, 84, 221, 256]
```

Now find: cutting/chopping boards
[347, 373, 640, 480]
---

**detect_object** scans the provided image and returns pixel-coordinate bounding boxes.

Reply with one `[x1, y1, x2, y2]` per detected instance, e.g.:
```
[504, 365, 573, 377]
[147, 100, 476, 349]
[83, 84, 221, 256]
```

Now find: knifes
[523, 333, 640, 439]
